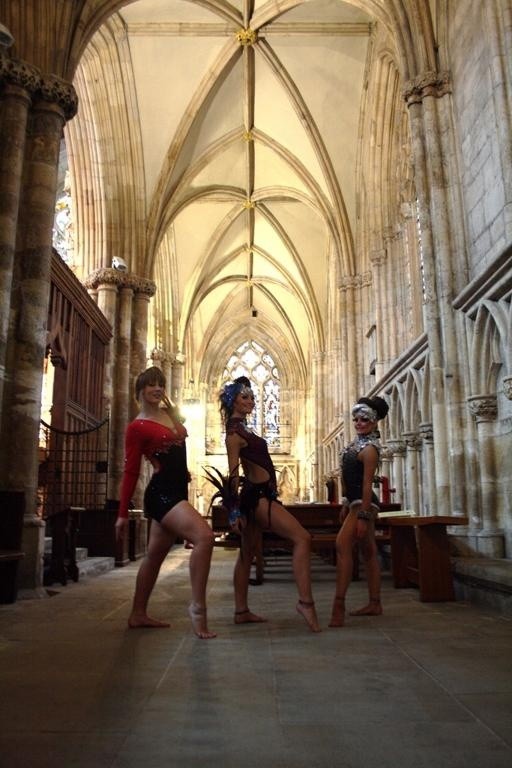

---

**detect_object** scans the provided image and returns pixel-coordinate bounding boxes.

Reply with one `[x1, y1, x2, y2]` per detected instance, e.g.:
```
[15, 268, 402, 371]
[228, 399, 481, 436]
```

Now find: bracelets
[357, 511, 371, 520]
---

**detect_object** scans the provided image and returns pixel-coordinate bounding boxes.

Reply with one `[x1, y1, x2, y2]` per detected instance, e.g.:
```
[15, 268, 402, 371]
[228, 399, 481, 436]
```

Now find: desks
[375, 514, 470, 603]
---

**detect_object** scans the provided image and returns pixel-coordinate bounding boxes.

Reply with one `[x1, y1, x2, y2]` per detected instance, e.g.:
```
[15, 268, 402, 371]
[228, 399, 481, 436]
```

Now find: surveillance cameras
[111, 256, 127, 272]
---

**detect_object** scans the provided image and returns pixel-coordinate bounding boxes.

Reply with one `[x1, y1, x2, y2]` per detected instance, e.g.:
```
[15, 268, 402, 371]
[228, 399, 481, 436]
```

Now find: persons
[220, 376, 321, 633]
[327, 396, 389, 628]
[115, 365, 218, 639]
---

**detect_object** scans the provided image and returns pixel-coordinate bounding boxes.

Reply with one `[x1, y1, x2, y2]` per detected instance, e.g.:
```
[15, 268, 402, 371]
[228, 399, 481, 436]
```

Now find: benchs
[253, 504, 401, 585]
